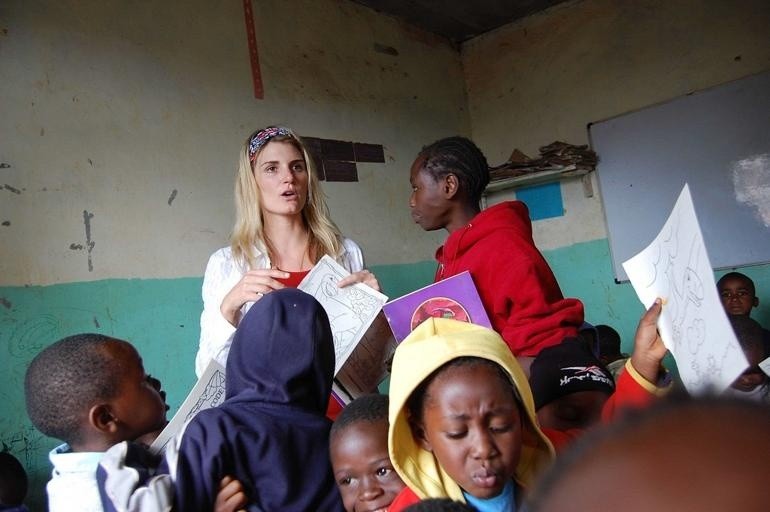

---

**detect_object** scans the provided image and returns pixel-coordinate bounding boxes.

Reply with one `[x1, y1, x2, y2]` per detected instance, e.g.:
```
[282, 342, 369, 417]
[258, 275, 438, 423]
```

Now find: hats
[527, 335, 617, 413]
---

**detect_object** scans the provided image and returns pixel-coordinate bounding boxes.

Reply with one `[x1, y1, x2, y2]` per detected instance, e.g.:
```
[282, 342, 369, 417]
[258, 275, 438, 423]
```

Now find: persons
[0, 271, 769, 512]
[195, 127, 381, 423]
[409, 135, 584, 381]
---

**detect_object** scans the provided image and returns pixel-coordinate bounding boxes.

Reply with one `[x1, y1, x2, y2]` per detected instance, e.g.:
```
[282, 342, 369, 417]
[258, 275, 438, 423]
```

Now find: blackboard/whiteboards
[587, 70, 770, 285]
